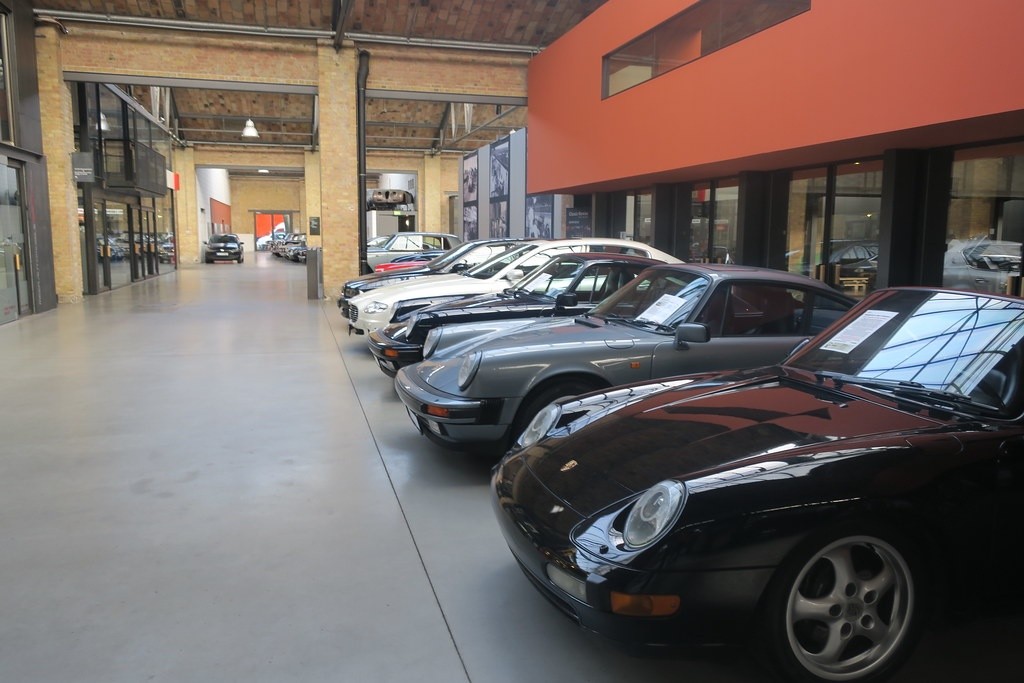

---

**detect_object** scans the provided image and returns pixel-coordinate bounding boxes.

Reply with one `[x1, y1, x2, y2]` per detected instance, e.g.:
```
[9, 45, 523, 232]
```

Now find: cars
[943, 240, 1022, 296]
[350, 238, 686, 328]
[202, 233, 245, 264]
[490, 287, 1024, 683]
[828, 241, 877, 289]
[367, 231, 465, 274]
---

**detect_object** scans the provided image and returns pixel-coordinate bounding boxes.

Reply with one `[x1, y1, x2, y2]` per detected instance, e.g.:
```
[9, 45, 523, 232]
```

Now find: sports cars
[393, 261, 862, 466]
[365, 252, 661, 375]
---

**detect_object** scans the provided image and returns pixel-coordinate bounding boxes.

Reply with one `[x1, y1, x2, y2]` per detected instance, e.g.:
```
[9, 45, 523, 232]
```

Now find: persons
[462, 165, 550, 240]
[947, 233, 960, 252]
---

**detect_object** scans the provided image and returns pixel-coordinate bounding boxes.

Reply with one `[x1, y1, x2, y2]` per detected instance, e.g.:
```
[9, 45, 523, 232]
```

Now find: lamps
[95, 113, 111, 131]
[241, 119, 260, 139]
